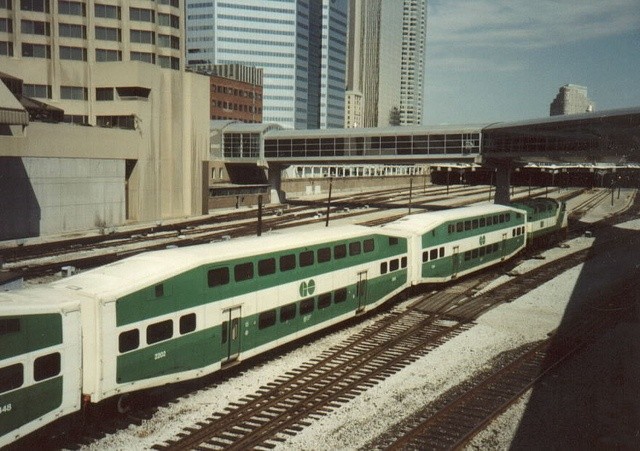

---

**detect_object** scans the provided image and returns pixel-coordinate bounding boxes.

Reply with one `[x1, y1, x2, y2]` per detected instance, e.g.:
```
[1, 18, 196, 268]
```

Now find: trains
[0, 196, 569, 451]
